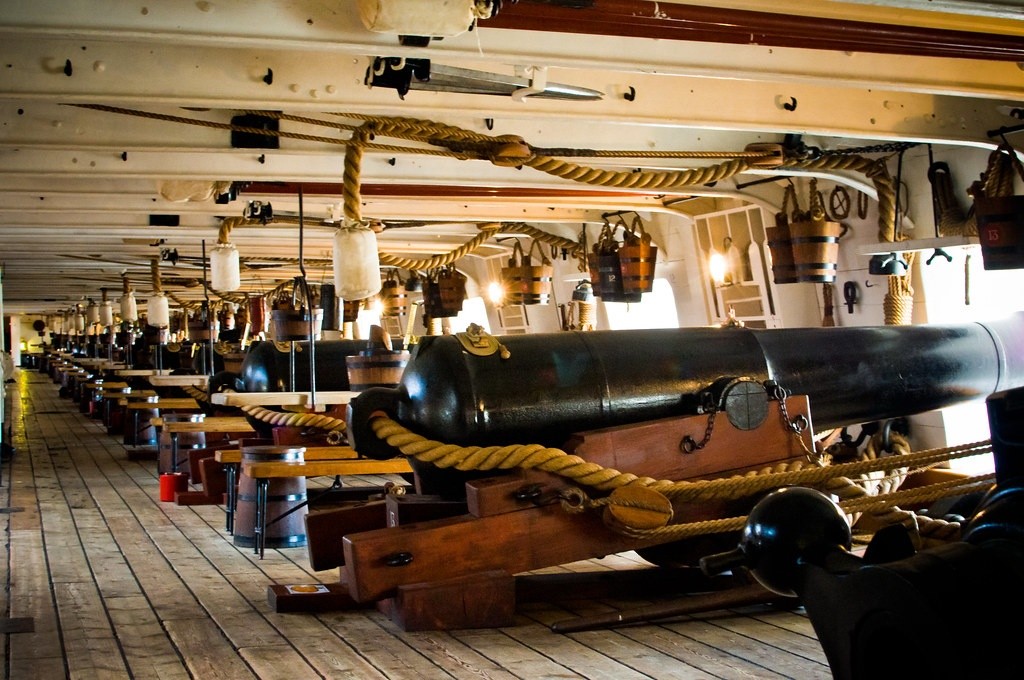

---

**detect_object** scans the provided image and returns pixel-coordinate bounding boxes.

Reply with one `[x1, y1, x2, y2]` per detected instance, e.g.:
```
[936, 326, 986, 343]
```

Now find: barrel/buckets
[766, 184, 797, 283]
[501, 241, 524, 305]
[973, 143, 1024, 270]
[788, 179, 840, 283]
[21, 267, 470, 549]
[618, 216, 658, 294]
[596, 220, 641, 304]
[586, 225, 602, 297]
[520, 239, 554, 305]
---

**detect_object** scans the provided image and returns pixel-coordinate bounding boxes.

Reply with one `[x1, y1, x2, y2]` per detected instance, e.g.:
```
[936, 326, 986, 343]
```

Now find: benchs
[126, 402, 199, 447]
[85, 381, 128, 419]
[243, 458, 413, 560]
[47, 359, 64, 364]
[75, 377, 90, 408]
[162, 417, 255, 472]
[58, 367, 83, 381]
[52, 363, 72, 369]
[63, 372, 88, 399]
[214, 446, 366, 536]
[150, 418, 166, 477]
[103, 392, 145, 436]
